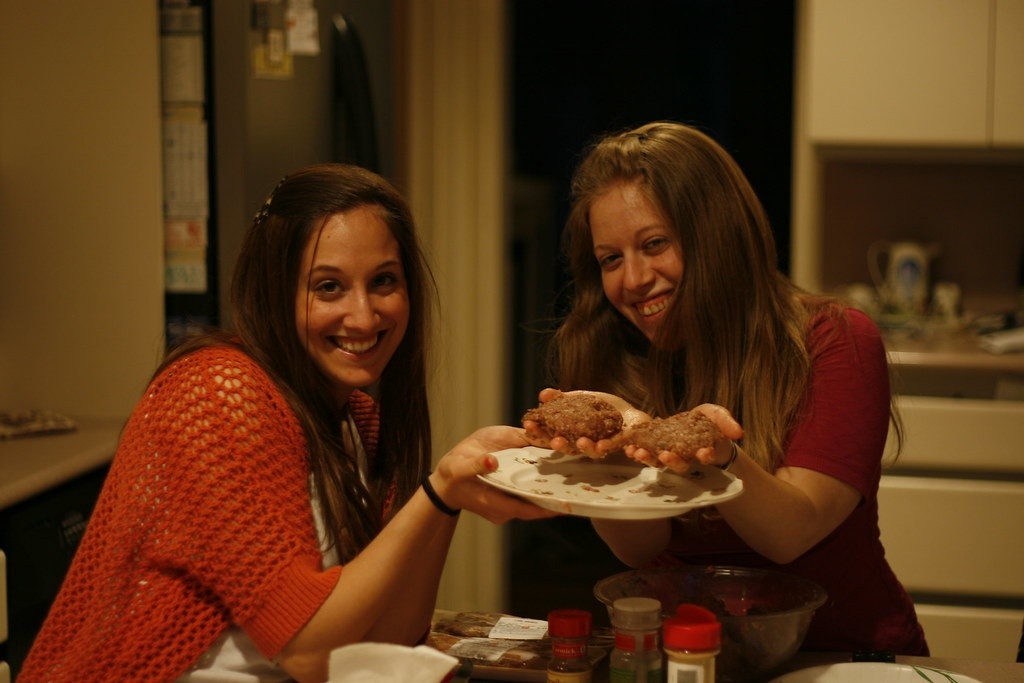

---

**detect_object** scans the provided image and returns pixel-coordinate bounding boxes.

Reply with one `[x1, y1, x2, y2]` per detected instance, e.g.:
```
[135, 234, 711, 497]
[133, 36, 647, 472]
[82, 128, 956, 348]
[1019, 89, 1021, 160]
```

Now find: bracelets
[720, 441, 737, 473]
[421, 471, 461, 517]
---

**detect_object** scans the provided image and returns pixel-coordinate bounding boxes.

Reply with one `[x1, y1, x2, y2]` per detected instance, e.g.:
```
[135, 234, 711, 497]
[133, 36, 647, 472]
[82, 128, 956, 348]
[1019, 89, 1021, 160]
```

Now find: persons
[14, 162, 579, 683]
[526, 122, 929, 666]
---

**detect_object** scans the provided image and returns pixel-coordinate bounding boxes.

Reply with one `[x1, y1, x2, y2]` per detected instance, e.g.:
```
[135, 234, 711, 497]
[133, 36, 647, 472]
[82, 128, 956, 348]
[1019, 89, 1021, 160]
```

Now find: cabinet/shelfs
[876, 318, 1024, 658]
[802, 2, 1022, 162]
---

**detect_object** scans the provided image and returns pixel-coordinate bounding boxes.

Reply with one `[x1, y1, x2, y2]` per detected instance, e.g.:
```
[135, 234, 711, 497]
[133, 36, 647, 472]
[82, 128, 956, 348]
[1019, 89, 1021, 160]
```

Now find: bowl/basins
[594, 564, 828, 674]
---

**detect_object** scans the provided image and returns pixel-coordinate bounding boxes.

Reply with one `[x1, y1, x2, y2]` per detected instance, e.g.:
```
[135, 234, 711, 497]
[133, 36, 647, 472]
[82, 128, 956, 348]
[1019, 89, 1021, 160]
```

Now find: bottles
[609, 597, 662, 683]
[663, 604, 721, 683]
[545, 610, 592, 683]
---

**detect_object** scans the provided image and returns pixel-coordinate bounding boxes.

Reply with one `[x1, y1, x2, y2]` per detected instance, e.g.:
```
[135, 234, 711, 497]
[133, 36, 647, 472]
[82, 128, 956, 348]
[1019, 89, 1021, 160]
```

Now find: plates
[764, 662, 981, 683]
[477, 447, 744, 520]
